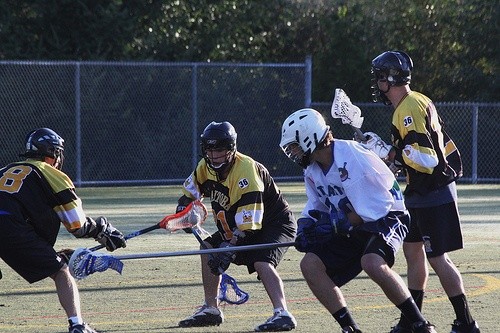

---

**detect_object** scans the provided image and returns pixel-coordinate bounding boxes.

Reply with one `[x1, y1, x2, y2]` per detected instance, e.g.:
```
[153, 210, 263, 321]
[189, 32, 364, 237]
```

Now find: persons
[0, 126, 128, 333]
[353, 50, 482, 333]
[175, 120, 298, 333]
[277, 106, 438, 333]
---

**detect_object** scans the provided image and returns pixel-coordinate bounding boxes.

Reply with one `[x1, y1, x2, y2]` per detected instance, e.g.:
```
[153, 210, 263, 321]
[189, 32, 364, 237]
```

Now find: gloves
[361, 132, 392, 160]
[302, 209, 349, 241]
[294, 217, 319, 253]
[80, 217, 98, 238]
[382, 157, 403, 174]
[94, 216, 126, 252]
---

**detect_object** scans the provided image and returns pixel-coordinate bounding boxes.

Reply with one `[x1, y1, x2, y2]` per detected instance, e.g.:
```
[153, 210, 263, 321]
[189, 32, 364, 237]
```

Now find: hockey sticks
[89, 198, 208, 252]
[68, 241, 295, 280]
[192, 227, 250, 306]
[330, 87, 367, 141]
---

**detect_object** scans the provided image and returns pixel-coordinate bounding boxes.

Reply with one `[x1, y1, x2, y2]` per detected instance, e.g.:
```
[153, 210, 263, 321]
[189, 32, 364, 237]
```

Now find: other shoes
[389, 320, 411, 333]
[449, 320, 480, 333]
[416, 321, 437, 333]
[342, 325, 362, 333]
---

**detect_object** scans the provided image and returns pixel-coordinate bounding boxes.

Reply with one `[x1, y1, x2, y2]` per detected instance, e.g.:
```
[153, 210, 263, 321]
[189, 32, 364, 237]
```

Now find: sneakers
[254, 308, 297, 332]
[68, 319, 97, 333]
[178, 306, 222, 328]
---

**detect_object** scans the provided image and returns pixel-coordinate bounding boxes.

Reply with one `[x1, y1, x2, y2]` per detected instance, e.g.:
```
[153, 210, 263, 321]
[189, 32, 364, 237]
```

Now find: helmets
[201, 121, 237, 174]
[280, 108, 330, 154]
[24, 128, 65, 172]
[372, 51, 414, 86]
